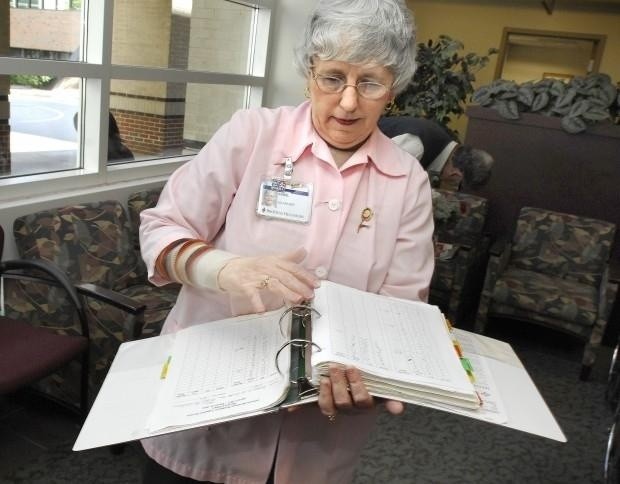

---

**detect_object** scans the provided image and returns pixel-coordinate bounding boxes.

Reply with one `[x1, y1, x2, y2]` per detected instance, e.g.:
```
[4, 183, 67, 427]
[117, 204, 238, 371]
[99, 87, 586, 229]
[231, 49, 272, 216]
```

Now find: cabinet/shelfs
[459, 100, 619, 279]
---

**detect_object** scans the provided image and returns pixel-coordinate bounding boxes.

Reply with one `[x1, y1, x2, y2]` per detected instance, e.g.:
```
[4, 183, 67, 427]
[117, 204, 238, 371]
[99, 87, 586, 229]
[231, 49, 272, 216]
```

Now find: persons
[136, 0, 439, 483]
[263, 190, 275, 207]
[376, 115, 494, 189]
[74, 106, 135, 166]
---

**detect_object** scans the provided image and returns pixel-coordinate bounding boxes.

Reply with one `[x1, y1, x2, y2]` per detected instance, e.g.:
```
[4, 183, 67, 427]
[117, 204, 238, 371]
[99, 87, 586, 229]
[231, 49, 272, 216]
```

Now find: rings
[321, 409, 337, 426]
[264, 274, 269, 289]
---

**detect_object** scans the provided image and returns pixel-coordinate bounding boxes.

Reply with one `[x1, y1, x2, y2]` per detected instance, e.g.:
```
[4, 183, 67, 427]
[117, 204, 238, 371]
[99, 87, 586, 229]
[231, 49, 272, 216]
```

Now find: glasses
[311, 67, 391, 100]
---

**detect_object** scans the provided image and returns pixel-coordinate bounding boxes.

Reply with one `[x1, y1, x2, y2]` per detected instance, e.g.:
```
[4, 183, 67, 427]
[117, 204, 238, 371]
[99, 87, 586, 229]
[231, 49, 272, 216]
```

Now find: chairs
[423, 188, 492, 329]
[6, 199, 181, 456]
[475, 206, 617, 381]
[127, 187, 181, 292]
[0, 230, 91, 427]
